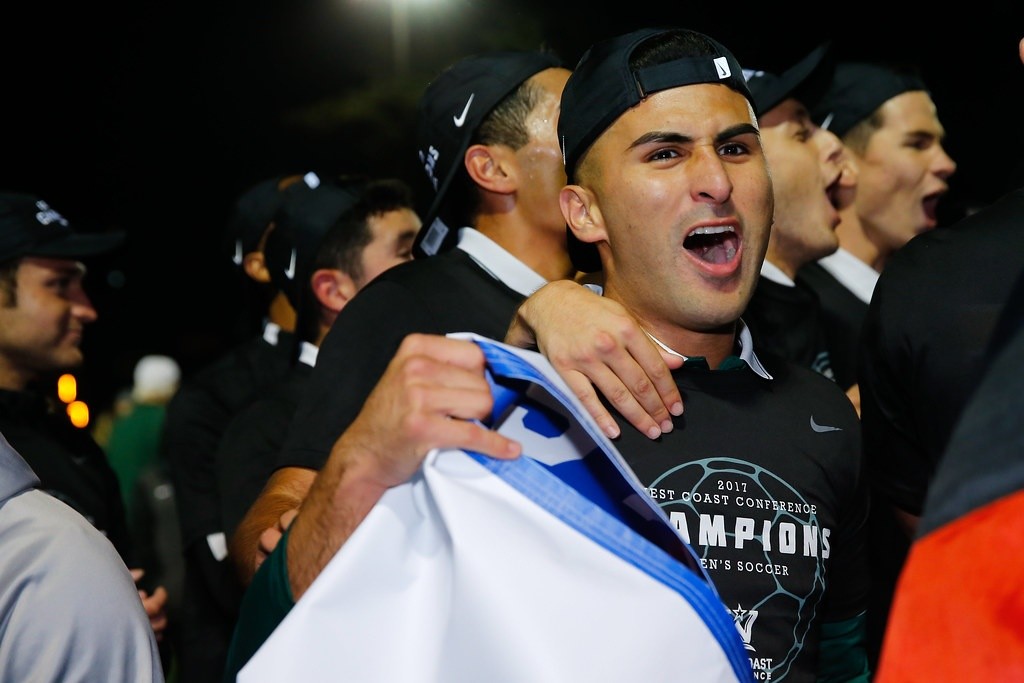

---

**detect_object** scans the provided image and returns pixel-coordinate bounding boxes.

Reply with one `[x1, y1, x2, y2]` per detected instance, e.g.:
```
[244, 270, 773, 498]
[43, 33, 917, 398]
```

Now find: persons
[0, 23, 1024, 683]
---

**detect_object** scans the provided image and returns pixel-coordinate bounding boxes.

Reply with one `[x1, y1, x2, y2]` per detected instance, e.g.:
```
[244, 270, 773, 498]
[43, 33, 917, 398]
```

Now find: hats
[264, 176, 383, 289]
[412, 49, 576, 261]
[0, 194, 127, 264]
[742, 46, 825, 118]
[557, 28, 759, 273]
[806, 64, 937, 138]
[221, 176, 315, 270]
